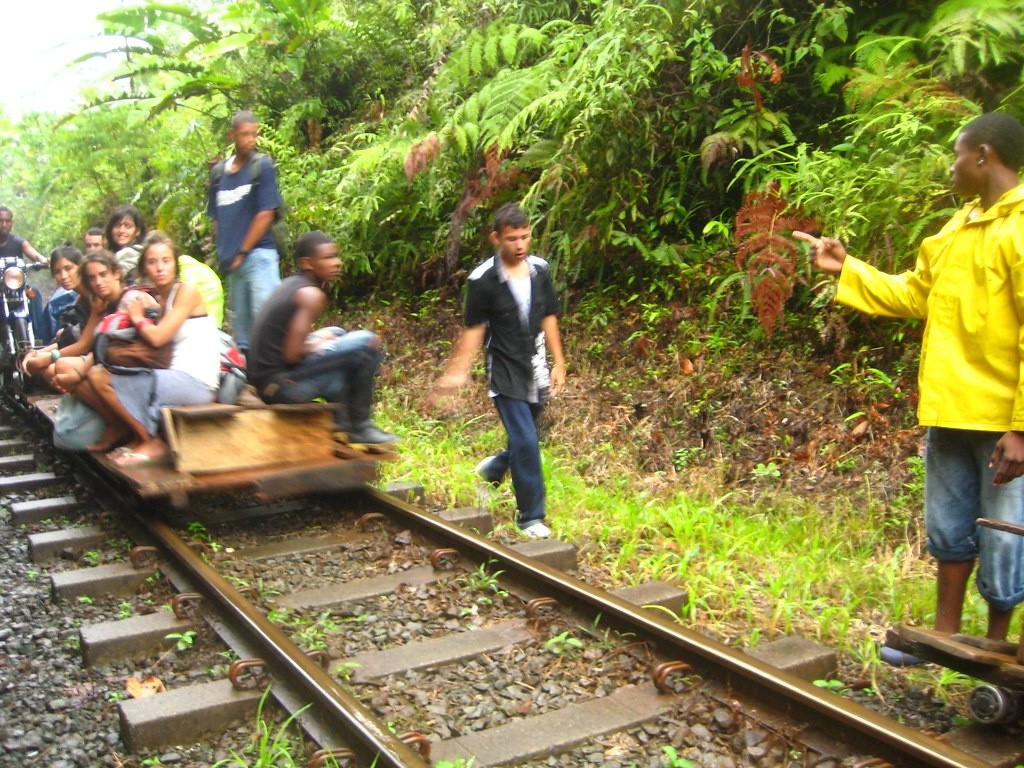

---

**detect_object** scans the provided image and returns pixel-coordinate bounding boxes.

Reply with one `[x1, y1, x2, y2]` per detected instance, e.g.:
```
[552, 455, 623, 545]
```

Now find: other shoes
[880, 642, 926, 667]
[334, 423, 398, 450]
[475, 455, 499, 504]
[523, 521, 550, 538]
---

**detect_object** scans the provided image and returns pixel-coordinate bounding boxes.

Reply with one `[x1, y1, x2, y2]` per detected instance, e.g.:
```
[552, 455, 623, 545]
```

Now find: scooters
[0, 255, 51, 410]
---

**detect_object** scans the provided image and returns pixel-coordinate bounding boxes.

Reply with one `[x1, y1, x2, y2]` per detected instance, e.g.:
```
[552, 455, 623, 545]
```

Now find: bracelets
[51, 350, 60, 362]
[238, 249, 248, 255]
[136, 320, 148, 331]
[81, 355, 86, 361]
[74, 368, 83, 379]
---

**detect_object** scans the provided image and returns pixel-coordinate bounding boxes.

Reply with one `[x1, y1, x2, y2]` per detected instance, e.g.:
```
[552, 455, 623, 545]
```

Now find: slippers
[86, 437, 132, 453]
[105, 447, 171, 469]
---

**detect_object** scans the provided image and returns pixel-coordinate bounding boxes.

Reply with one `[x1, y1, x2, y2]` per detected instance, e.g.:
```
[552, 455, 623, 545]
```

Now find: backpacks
[214, 155, 289, 260]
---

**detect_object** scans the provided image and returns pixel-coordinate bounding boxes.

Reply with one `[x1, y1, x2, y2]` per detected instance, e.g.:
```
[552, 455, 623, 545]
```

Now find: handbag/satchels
[106, 336, 174, 371]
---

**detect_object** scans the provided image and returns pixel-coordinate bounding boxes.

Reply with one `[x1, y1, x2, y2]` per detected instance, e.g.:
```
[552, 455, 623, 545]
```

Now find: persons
[21, 206, 223, 465]
[428, 205, 567, 540]
[0, 206, 50, 347]
[207, 111, 283, 355]
[246, 230, 401, 454]
[791, 111, 1024, 666]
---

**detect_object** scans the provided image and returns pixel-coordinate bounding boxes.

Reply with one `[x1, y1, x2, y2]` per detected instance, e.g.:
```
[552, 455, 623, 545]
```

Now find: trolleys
[883, 515, 1024, 731]
